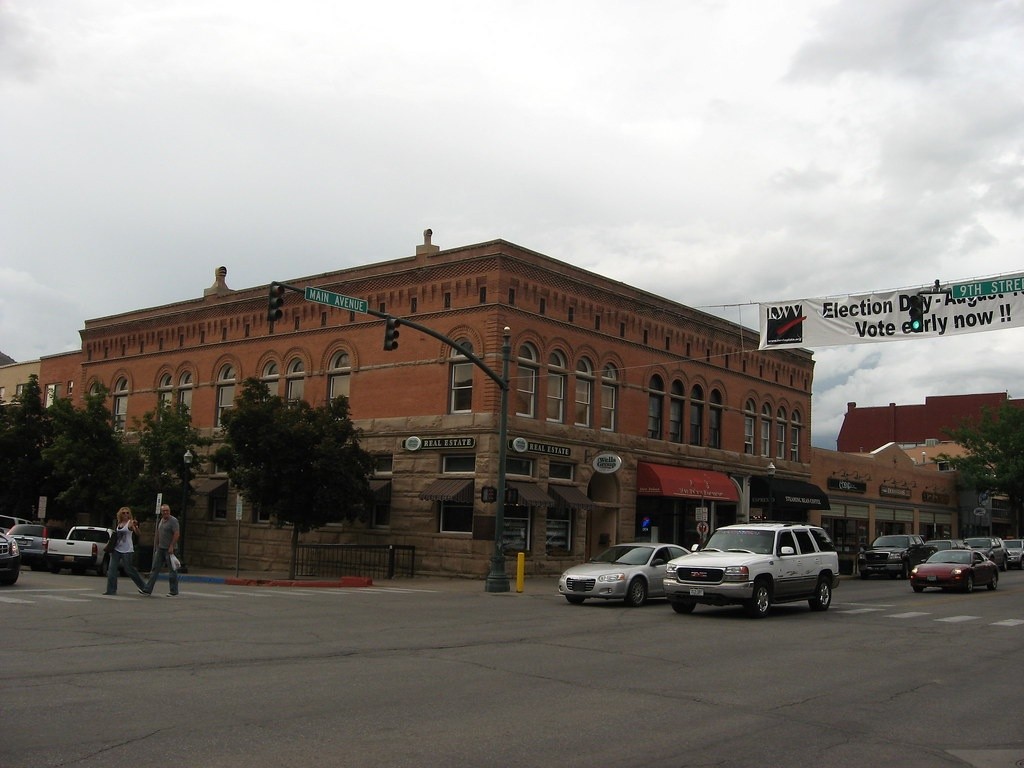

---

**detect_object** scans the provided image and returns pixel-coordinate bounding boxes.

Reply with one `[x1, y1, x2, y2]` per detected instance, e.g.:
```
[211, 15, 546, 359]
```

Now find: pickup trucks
[43, 525, 128, 578]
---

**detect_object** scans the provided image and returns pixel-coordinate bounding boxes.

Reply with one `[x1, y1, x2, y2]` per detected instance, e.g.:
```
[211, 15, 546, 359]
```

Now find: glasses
[122, 512, 129, 514]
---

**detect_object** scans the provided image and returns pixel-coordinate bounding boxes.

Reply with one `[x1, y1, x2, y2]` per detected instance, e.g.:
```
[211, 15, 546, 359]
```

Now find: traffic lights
[383, 317, 400, 351]
[907, 295, 924, 333]
[266, 281, 286, 322]
[480, 485, 497, 503]
[505, 488, 518, 505]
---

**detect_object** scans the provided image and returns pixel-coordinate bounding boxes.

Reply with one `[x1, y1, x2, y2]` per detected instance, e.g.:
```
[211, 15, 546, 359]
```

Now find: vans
[0, 514, 34, 535]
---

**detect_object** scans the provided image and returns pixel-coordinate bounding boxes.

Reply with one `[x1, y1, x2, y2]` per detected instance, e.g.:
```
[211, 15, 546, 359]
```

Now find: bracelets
[134, 525, 138, 530]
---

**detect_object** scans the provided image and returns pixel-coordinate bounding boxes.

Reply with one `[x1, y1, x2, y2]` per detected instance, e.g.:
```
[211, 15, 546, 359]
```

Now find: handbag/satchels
[103, 531, 117, 553]
[170, 554, 181, 571]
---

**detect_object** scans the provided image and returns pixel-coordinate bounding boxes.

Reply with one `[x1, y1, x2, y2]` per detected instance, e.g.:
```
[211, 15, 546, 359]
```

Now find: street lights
[175, 449, 189, 575]
[766, 462, 777, 521]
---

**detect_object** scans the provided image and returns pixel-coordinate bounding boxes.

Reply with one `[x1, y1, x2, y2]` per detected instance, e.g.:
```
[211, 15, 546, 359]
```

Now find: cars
[558, 541, 692, 608]
[928, 535, 1024, 571]
[5, 524, 67, 571]
[909, 548, 999, 594]
[0, 528, 21, 587]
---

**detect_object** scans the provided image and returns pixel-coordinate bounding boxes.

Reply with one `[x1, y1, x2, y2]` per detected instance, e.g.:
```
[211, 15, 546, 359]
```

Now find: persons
[138, 504, 179, 597]
[102, 507, 147, 595]
[963, 541, 972, 550]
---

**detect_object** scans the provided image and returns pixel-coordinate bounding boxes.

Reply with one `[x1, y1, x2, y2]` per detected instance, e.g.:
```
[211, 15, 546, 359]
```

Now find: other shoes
[139, 592, 149, 596]
[138, 589, 151, 595]
[102, 591, 116, 595]
[166, 592, 179, 597]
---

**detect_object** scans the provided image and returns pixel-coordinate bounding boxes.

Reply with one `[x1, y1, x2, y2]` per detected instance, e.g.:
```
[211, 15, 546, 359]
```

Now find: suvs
[856, 532, 934, 580]
[662, 519, 841, 619]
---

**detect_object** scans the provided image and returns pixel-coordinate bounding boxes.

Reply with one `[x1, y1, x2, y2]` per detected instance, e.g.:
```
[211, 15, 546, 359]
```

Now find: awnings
[366, 480, 391, 503]
[638, 462, 741, 503]
[547, 484, 595, 511]
[749, 475, 831, 510]
[189, 478, 227, 496]
[504, 481, 554, 507]
[418, 479, 473, 503]
